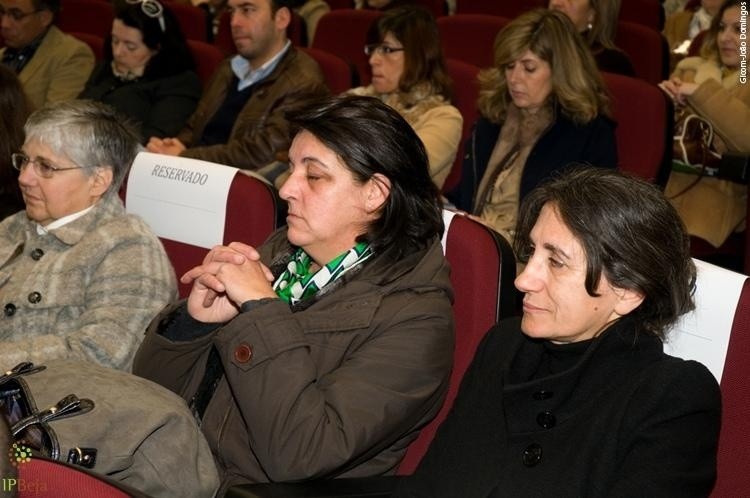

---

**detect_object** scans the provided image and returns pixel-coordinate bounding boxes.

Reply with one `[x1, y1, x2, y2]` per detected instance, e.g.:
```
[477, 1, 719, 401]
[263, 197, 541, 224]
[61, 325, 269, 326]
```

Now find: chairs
[0, 1, 750, 498]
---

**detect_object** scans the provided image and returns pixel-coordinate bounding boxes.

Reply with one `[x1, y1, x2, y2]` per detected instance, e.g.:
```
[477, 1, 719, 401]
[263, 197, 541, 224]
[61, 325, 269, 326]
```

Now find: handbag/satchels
[674, 113, 722, 168]
[0, 362, 220, 498]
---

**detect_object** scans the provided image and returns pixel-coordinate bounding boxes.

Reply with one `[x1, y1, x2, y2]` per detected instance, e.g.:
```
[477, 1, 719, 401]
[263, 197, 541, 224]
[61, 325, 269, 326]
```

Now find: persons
[130, 93, 455, 498]
[410, 166, 724, 497]
[1, 99, 182, 380]
[1, 0, 748, 272]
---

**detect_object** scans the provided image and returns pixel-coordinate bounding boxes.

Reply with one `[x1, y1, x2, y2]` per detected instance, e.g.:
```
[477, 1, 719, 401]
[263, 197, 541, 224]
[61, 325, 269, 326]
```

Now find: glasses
[365, 43, 406, 56]
[126, 0, 165, 32]
[11, 153, 94, 178]
